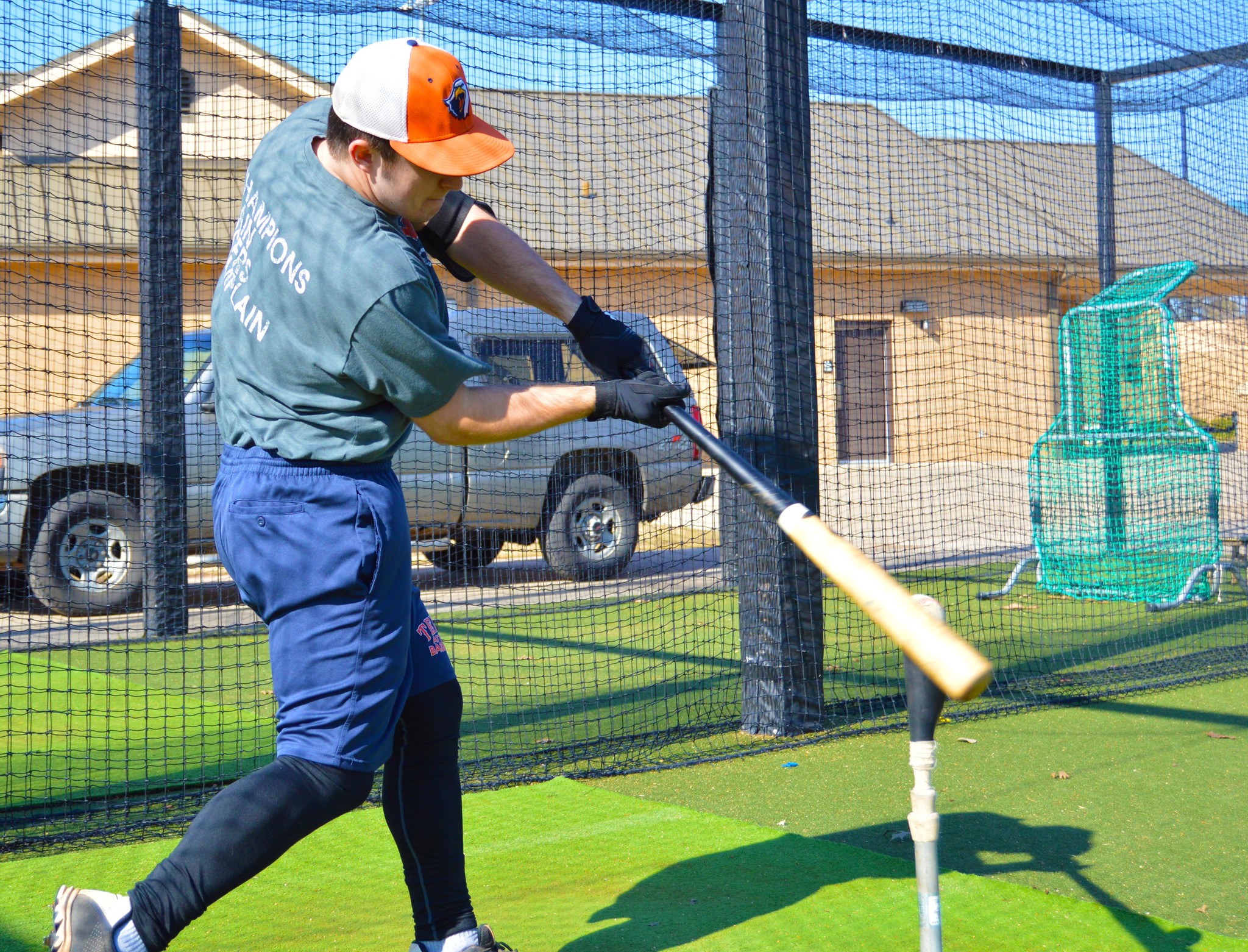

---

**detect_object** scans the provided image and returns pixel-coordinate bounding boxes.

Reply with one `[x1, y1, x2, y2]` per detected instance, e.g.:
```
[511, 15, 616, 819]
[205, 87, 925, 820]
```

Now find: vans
[1, 301, 719, 622]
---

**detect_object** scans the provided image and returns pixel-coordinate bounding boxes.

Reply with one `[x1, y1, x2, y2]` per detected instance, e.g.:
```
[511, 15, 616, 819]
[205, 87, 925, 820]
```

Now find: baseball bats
[623, 360, 996, 705]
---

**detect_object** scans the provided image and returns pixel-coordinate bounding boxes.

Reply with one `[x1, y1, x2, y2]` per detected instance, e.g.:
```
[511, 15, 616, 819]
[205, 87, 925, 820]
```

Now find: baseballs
[912, 593, 943, 618]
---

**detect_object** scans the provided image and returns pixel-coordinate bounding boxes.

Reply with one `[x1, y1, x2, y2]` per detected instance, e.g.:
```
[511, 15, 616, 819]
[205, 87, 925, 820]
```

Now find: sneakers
[49, 883, 132, 952]
[409, 923, 519, 952]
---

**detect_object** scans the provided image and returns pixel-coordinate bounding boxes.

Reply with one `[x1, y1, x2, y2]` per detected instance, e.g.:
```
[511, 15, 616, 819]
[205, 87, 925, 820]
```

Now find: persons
[38, 31, 704, 950]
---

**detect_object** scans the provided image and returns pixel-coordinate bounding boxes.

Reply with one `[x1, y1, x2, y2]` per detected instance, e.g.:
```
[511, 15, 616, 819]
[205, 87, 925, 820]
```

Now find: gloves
[586, 371, 691, 429]
[562, 296, 655, 381]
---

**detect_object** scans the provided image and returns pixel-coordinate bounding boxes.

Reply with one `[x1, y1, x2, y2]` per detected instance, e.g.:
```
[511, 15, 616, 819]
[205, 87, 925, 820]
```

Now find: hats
[331, 36, 516, 177]
